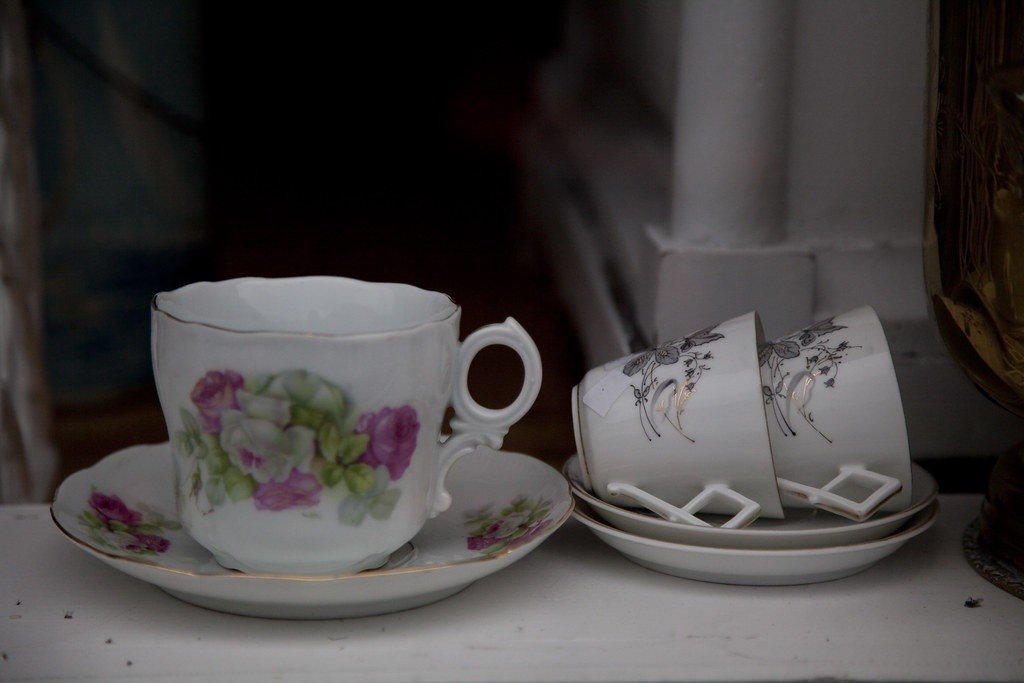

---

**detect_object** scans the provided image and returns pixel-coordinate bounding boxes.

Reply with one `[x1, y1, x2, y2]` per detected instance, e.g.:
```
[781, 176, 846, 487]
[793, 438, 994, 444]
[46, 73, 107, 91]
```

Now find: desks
[0, 494, 1024, 683]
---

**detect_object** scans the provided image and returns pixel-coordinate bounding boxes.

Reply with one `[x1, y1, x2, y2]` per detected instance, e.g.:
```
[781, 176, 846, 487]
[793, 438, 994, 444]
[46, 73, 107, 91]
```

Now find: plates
[562, 452, 938, 585]
[49, 443, 575, 622]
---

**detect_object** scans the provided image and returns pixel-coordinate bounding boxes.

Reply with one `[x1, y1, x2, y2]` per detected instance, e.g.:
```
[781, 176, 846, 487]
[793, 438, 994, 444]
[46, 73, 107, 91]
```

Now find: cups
[757, 306, 919, 521]
[571, 311, 786, 527]
[151, 274, 545, 579]
